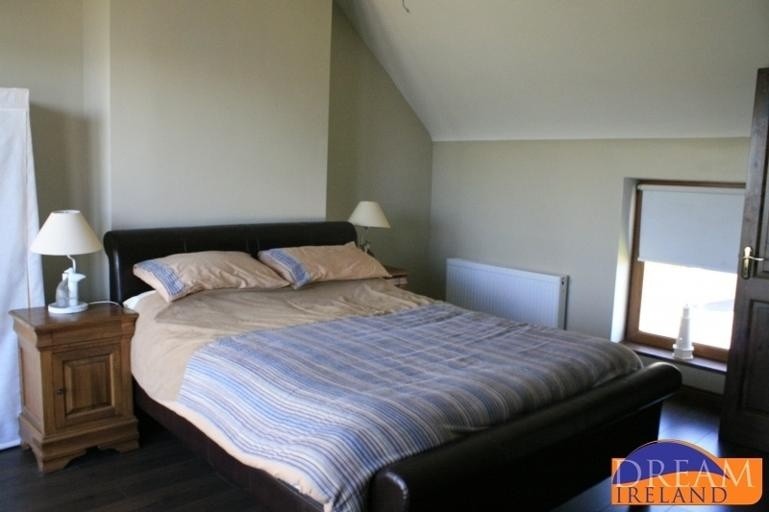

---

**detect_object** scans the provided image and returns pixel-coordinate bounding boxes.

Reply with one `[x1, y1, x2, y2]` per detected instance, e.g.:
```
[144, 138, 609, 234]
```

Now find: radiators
[443, 256, 569, 331]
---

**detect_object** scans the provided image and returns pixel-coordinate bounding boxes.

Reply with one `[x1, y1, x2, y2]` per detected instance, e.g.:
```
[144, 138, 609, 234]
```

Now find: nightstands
[8, 302, 142, 475]
[377, 263, 409, 287]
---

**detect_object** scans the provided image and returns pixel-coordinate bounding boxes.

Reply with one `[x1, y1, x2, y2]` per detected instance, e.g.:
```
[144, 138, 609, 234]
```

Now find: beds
[102, 219, 683, 512]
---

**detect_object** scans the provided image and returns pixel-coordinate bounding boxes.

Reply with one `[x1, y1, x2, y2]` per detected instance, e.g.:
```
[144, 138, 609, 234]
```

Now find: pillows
[130, 249, 289, 303]
[258, 239, 392, 290]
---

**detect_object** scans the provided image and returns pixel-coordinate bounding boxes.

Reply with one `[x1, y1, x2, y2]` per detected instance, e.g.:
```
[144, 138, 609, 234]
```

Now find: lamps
[29, 209, 104, 315]
[347, 201, 391, 253]
